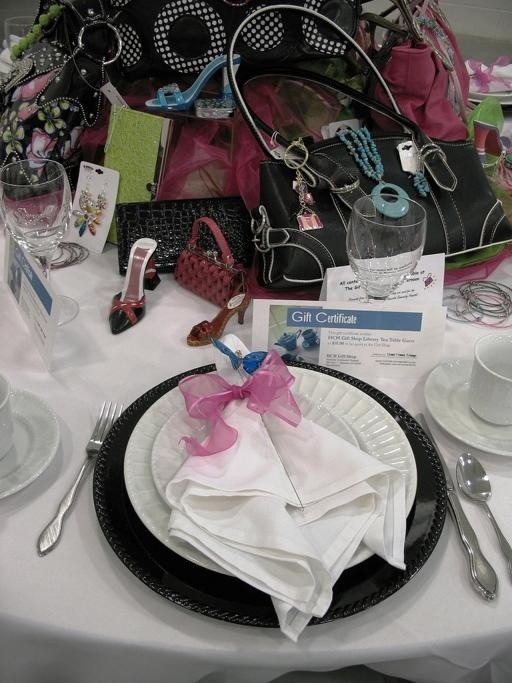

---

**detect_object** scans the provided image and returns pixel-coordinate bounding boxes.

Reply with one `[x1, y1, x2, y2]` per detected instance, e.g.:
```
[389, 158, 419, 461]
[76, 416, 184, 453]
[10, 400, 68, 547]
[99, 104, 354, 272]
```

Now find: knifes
[413, 414, 498, 603]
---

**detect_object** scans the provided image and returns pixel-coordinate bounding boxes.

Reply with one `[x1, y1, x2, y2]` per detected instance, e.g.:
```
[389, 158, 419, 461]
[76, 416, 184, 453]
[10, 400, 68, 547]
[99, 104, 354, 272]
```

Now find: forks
[38, 400, 124, 554]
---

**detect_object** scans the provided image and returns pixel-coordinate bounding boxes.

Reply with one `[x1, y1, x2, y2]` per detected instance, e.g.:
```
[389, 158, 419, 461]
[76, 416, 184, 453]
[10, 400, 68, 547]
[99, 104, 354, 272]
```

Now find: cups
[468, 332, 512, 425]
[0, 158, 79, 329]
[345, 193, 427, 305]
[1, 375, 14, 460]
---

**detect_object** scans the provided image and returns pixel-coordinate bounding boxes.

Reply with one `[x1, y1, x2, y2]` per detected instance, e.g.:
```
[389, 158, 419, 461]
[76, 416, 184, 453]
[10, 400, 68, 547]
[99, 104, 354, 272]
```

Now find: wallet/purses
[114, 198, 253, 277]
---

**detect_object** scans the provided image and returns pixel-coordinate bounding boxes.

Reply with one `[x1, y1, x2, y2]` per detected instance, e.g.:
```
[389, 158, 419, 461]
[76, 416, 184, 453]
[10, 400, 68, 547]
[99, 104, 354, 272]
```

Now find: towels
[165, 333, 407, 643]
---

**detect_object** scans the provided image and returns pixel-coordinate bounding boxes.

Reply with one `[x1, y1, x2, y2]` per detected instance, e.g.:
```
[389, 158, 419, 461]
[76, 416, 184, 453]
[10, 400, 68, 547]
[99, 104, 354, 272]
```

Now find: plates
[124, 364, 416, 576]
[424, 358, 512, 458]
[93, 362, 449, 628]
[1, 394, 60, 502]
[149, 383, 360, 513]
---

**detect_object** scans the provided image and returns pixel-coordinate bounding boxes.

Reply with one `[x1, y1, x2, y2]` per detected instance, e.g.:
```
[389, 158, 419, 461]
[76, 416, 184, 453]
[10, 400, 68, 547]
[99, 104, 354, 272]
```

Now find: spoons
[456, 452, 511, 568]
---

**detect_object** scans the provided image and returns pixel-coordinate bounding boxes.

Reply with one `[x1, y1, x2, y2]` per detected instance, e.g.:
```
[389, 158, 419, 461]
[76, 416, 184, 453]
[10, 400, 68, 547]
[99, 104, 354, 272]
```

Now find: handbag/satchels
[174, 217, 249, 308]
[228, 3, 512, 290]
[354, 2, 474, 149]
[0, 1, 123, 200]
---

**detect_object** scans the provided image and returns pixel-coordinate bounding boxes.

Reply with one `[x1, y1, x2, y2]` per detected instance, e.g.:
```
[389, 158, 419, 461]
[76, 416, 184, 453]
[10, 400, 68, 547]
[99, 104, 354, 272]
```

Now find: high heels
[144, 53, 242, 112]
[107, 238, 159, 335]
[186, 291, 252, 348]
[470, 95, 507, 160]
